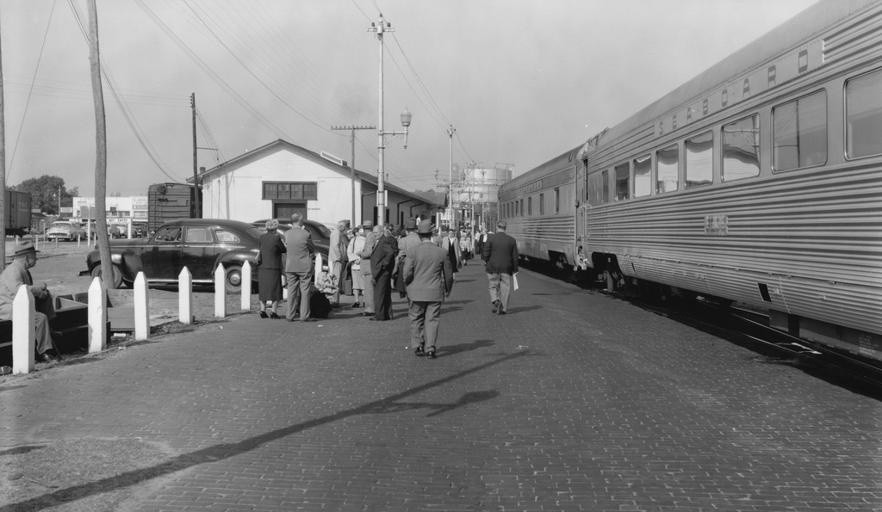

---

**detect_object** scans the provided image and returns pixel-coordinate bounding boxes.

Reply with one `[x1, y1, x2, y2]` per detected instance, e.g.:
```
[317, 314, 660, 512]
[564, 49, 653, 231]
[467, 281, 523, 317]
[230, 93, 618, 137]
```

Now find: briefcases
[340, 279, 355, 297]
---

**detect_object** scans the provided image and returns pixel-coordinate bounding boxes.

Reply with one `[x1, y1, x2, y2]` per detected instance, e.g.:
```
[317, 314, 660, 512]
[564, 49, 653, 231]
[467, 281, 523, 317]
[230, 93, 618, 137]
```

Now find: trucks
[149, 182, 202, 237]
[4, 190, 32, 237]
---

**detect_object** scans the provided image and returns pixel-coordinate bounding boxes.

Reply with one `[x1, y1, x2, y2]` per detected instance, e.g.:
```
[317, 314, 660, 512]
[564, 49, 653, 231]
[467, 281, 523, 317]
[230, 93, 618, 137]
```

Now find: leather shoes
[414, 346, 424, 357]
[427, 350, 438, 360]
[362, 312, 379, 322]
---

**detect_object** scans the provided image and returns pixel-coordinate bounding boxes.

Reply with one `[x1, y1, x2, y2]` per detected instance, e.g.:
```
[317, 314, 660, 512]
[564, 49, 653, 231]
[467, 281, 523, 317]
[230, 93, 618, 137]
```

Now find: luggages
[296, 284, 332, 318]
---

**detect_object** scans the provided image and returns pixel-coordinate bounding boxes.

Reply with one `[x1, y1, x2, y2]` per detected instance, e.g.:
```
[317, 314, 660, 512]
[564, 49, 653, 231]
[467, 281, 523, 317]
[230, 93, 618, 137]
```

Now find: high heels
[269, 311, 280, 319]
[258, 310, 270, 319]
[363, 300, 366, 307]
[351, 302, 361, 308]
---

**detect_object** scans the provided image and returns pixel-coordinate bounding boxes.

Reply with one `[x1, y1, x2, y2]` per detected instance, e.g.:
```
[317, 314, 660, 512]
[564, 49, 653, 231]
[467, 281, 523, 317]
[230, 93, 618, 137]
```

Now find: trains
[495, 0, 881, 358]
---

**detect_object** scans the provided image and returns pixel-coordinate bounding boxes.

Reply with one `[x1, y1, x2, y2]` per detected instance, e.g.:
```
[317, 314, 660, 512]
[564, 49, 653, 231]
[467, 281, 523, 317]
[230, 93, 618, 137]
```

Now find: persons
[0, 240, 60, 362]
[259, 214, 520, 359]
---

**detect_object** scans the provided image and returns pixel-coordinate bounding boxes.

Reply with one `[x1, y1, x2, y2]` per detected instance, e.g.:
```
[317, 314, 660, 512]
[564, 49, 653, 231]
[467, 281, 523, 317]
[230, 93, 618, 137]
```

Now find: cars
[42, 215, 149, 239]
[258, 218, 337, 246]
[248, 223, 331, 287]
[75, 219, 270, 295]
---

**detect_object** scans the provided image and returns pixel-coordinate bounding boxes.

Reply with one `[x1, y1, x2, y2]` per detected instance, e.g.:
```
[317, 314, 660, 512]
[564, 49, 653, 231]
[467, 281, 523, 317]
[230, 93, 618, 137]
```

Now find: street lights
[376, 108, 412, 228]
[470, 191, 484, 250]
[448, 167, 467, 231]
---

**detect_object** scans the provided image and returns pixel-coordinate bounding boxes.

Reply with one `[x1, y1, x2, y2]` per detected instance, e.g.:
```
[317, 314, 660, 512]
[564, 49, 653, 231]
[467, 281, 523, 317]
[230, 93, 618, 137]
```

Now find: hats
[405, 217, 418, 230]
[7, 240, 43, 257]
[362, 219, 374, 230]
[415, 220, 436, 235]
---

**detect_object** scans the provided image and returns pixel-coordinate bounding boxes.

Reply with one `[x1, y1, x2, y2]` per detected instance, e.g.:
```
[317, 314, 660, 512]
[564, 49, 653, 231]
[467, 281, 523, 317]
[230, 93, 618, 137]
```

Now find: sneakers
[40, 350, 55, 364]
[489, 300, 513, 315]
[299, 316, 319, 322]
[286, 315, 296, 323]
